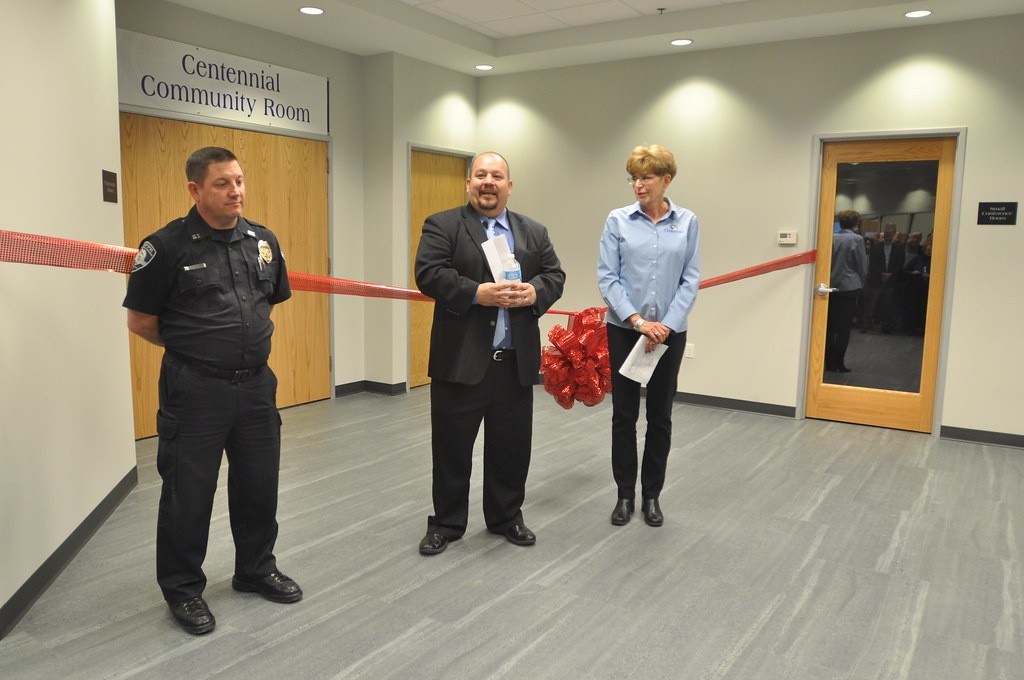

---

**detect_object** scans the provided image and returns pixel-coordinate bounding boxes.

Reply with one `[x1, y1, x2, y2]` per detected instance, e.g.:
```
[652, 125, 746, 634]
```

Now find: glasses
[626, 172, 658, 185]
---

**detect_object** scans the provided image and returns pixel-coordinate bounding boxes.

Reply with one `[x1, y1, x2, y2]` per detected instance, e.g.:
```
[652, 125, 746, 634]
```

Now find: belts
[490, 349, 516, 362]
[165, 347, 266, 384]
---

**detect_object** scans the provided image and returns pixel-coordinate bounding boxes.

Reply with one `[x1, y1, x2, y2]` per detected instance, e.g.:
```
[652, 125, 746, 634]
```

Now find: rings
[525, 299, 528, 303]
[651, 340, 654, 344]
[654, 332, 659, 336]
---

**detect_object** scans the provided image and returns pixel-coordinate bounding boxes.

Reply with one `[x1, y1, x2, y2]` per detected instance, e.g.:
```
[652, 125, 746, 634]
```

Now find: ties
[486, 218, 506, 346]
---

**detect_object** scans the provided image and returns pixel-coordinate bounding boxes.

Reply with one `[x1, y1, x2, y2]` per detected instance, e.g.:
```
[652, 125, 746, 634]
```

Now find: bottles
[503, 254, 522, 286]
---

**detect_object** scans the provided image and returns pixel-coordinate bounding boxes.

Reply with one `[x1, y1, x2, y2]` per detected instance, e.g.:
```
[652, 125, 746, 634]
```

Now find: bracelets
[634, 319, 644, 330]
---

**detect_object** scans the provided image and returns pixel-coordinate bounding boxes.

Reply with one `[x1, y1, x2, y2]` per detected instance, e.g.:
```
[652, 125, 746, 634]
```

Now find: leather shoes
[419, 530, 450, 555]
[232, 570, 303, 603]
[641, 495, 664, 526]
[167, 597, 216, 635]
[826, 366, 852, 373]
[503, 517, 537, 546]
[610, 496, 636, 526]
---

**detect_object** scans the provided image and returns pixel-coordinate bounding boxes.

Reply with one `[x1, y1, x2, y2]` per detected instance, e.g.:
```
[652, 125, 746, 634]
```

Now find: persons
[596, 144, 699, 527]
[853, 223, 933, 337]
[123, 148, 303, 634]
[824, 210, 867, 372]
[414, 152, 566, 555]
